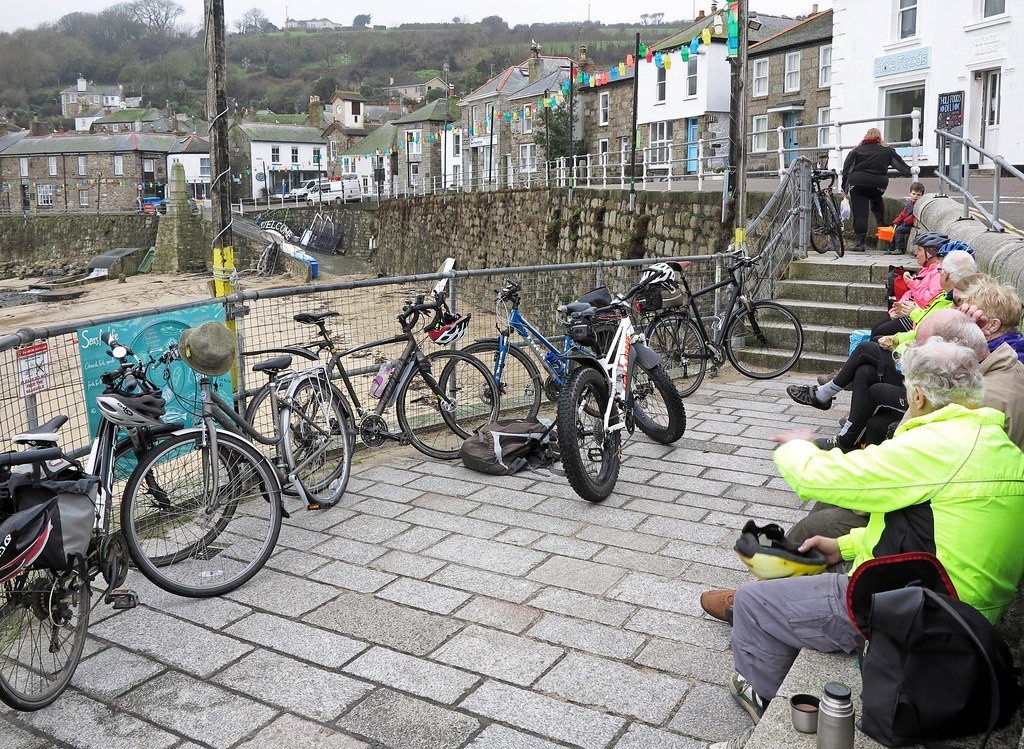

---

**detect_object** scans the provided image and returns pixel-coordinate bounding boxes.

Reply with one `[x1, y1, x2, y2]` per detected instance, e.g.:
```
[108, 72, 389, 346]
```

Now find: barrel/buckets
[877, 224, 897, 242]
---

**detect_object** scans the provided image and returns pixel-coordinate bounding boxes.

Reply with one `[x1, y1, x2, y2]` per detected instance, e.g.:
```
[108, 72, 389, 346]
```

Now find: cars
[153, 197, 197, 215]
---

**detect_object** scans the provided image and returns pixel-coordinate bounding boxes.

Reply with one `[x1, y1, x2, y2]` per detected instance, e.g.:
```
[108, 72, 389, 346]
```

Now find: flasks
[816, 682, 856, 749]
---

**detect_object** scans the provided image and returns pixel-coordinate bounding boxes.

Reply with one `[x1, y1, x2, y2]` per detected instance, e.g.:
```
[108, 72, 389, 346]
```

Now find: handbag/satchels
[848, 330, 871, 359]
[9, 474, 101, 578]
[839, 195, 850, 220]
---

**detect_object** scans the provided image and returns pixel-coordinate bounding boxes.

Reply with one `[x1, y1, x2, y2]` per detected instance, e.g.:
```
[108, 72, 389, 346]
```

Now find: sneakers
[839, 417, 853, 426]
[787, 383, 837, 411]
[704, 725, 757, 749]
[811, 434, 855, 457]
[729, 670, 770, 725]
[701, 589, 738, 624]
[817, 369, 840, 385]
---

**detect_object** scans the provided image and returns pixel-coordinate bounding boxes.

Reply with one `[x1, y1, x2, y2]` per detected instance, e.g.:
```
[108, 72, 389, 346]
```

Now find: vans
[307, 180, 361, 206]
[288, 177, 330, 202]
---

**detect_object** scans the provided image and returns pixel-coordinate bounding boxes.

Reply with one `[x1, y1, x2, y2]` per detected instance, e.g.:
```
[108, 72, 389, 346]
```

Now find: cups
[370, 363, 392, 398]
[545, 352, 564, 373]
[789, 693, 821, 734]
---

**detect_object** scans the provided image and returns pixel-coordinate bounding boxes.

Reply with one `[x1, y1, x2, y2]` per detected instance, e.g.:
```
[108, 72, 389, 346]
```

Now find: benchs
[745, 647, 1024, 749]
[647, 168, 675, 182]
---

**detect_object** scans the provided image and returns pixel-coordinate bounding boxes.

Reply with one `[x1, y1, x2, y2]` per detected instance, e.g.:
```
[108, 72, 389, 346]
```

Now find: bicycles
[793, 153, 846, 257]
[437, 276, 619, 442]
[553, 268, 687, 503]
[636, 248, 804, 399]
[119, 352, 352, 599]
[0, 330, 241, 712]
[242, 294, 501, 496]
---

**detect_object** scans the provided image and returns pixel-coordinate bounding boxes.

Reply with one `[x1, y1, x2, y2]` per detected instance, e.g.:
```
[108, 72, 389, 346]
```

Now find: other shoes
[883, 249, 895, 255]
[891, 249, 905, 255]
[850, 244, 865, 252]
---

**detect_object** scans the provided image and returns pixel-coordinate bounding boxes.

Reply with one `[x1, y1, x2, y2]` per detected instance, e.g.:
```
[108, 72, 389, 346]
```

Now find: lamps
[975, 72, 982, 80]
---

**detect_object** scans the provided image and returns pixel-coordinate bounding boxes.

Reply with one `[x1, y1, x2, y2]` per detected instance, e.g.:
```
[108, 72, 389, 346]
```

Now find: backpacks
[634, 282, 684, 313]
[571, 285, 616, 317]
[461, 416, 565, 475]
[845, 551, 1018, 749]
[884, 265, 918, 312]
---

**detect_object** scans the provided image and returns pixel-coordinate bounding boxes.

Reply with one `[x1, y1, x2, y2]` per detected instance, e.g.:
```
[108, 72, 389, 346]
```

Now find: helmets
[427, 312, 472, 347]
[936, 241, 976, 263]
[638, 261, 676, 285]
[910, 231, 950, 253]
[733, 519, 826, 582]
[0, 495, 62, 584]
[95, 382, 168, 427]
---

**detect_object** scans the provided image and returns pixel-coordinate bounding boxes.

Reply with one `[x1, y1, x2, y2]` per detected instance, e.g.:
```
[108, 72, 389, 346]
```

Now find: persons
[883, 182, 925, 255]
[700, 303, 1024, 625]
[728, 335, 1024, 726]
[785, 231, 1024, 452]
[841, 128, 911, 252]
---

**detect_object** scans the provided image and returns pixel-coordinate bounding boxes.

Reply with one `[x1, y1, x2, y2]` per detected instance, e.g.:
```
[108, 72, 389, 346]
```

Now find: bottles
[93, 484, 106, 528]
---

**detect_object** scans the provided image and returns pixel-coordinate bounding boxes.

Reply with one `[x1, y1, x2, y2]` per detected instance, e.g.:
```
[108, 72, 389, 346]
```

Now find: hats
[178, 320, 236, 377]
